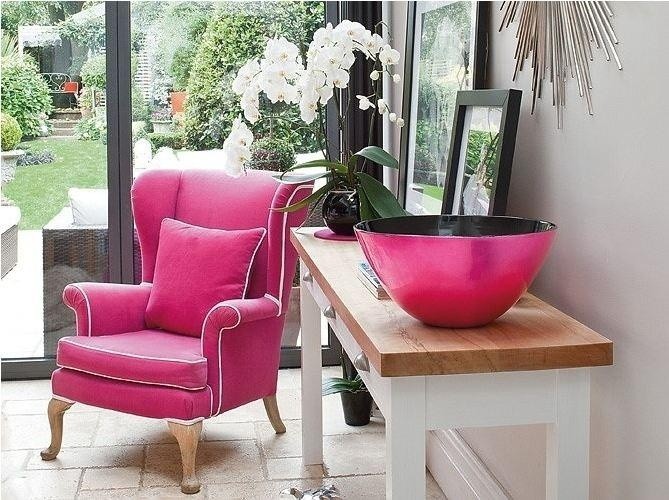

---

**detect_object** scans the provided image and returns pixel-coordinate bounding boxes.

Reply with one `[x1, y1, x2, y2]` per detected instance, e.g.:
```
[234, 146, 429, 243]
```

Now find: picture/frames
[395, 1, 522, 215]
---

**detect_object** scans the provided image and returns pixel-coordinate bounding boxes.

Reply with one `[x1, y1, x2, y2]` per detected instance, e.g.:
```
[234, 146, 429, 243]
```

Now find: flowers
[218, 18, 405, 180]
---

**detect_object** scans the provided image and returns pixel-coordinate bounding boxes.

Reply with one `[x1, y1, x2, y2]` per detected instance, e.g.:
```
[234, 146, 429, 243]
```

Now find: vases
[320, 188, 375, 234]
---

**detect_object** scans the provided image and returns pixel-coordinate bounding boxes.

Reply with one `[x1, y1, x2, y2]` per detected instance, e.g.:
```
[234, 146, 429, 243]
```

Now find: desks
[289, 212, 613, 500]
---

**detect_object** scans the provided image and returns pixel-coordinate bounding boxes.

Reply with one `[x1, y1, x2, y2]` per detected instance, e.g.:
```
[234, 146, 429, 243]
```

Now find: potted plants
[321, 366, 372, 426]
[132, 105, 173, 136]
[1, 113, 28, 186]
[77, 86, 102, 117]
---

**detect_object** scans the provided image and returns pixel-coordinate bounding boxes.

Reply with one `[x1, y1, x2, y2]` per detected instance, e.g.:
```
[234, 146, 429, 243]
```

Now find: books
[355, 273, 391, 300]
[353, 266, 388, 293]
[354, 259, 383, 289]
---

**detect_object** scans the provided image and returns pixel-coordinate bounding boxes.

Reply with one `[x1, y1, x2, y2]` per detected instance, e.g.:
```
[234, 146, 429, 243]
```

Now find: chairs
[38, 167, 314, 492]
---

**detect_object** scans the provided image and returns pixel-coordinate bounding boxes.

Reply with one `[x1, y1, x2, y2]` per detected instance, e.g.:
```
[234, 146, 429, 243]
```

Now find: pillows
[67, 184, 108, 226]
[144, 219, 271, 338]
